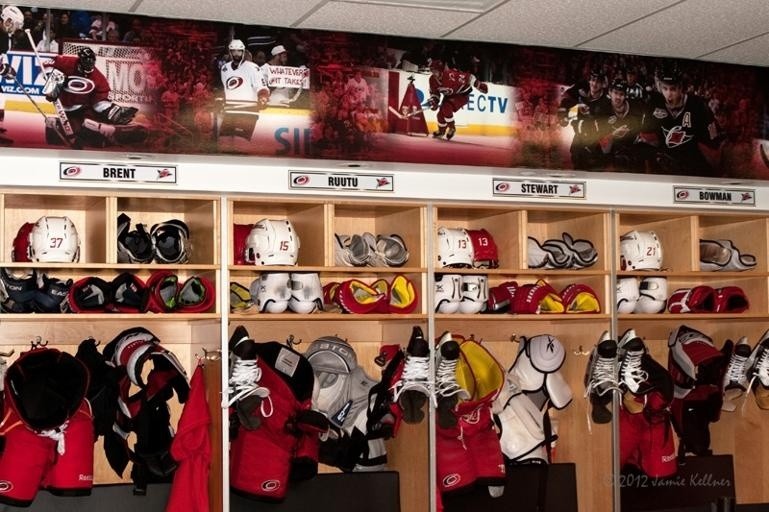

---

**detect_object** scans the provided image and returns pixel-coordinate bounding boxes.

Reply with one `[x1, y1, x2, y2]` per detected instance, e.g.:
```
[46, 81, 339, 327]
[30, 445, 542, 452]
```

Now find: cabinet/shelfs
[614, 209, 769, 512]
[225, 196, 433, 512]
[430, 200, 612, 512]
[2, 191, 221, 512]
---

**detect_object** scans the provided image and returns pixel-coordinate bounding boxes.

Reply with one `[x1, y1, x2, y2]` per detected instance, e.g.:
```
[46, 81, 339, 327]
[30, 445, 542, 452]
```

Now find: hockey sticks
[388, 105, 432, 119]
[215, 88, 302, 104]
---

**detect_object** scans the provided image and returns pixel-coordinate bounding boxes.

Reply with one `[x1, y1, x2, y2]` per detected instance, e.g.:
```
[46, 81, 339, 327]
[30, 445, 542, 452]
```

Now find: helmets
[1, 6, 24, 29]
[430, 60, 443, 76]
[620, 230, 663, 271]
[13, 216, 80, 263]
[438, 226, 499, 269]
[78, 47, 95, 70]
[589, 62, 683, 92]
[228, 40, 245, 65]
[244, 219, 300, 266]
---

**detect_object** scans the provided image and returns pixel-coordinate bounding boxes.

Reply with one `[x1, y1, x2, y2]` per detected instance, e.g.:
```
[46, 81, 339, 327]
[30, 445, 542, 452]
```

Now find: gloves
[477, 81, 488, 93]
[429, 96, 439, 110]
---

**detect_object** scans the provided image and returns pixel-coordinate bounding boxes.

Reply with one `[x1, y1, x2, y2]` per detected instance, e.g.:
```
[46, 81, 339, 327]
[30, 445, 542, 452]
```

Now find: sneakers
[590, 338, 654, 423]
[433, 129, 446, 136]
[721, 338, 769, 412]
[397, 338, 459, 429]
[228, 336, 273, 417]
[447, 128, 455, 138]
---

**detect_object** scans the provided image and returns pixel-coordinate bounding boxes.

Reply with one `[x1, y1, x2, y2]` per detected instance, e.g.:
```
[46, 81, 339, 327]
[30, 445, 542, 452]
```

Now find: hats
[271, 45, 286, 56]
